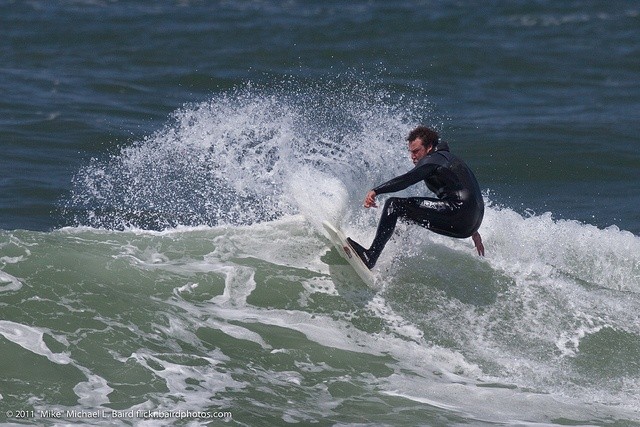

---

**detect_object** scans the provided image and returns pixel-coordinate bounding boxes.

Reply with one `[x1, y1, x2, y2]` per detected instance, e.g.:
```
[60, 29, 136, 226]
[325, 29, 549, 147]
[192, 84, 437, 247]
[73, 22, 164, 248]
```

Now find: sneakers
[346, 237, 375, 270]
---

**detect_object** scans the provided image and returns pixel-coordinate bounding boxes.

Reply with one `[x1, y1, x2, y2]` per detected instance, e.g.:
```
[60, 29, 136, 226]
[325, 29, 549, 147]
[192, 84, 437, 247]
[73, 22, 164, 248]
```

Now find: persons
[345, 125, 486, 270]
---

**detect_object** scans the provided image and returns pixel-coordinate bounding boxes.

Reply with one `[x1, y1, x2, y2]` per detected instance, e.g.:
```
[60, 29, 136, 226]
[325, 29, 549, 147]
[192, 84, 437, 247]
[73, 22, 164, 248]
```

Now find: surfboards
[322, 220, 378, 288]
